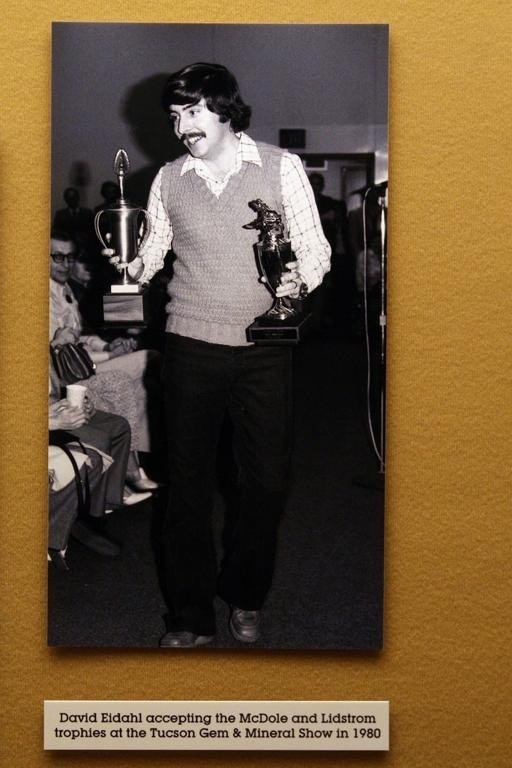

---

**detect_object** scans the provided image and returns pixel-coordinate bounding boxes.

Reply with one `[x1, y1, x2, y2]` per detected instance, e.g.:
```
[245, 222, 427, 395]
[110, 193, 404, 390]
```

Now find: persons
[48, 182, 166, 571]
[309, 174, 382, 329]
[100, 62, 333, 648]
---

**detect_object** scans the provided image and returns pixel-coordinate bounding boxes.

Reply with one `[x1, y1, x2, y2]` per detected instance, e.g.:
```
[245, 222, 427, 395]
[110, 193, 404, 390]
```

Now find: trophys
[94, 149, 153, 328]
[242, 199, 313, 346]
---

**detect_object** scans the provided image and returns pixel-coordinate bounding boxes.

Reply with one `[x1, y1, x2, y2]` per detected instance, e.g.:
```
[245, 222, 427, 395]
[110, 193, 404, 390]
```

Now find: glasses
[50, 253, 78, 263]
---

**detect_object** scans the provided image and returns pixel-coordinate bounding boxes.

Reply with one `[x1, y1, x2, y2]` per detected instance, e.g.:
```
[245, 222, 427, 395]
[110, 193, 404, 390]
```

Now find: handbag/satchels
[49, 429, 81, 449]
[51, 340, 96, 384]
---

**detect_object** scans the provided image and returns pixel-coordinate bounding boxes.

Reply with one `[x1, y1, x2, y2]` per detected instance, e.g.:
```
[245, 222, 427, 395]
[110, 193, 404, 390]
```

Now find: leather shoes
[128, 467, 160, 491]
[228, 606, 263, 644]
[103, 485, 153, 515]
[159, 630, 216, 649]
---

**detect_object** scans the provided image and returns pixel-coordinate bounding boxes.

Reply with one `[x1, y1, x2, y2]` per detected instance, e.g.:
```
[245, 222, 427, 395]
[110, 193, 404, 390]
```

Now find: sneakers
[70, 519, 122, 559]
